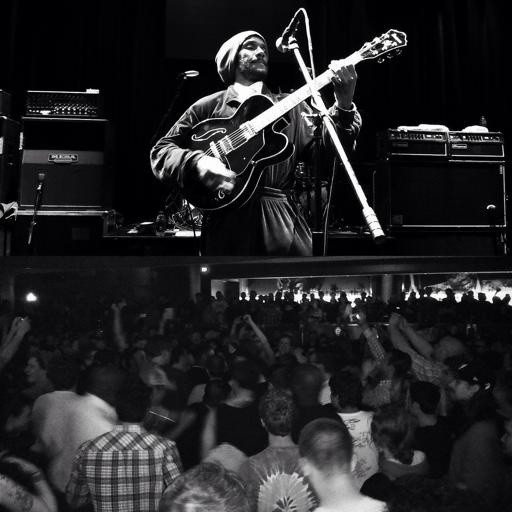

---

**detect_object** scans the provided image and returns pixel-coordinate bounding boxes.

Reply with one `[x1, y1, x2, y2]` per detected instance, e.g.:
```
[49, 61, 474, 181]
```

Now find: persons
[147, 31, 362, 257]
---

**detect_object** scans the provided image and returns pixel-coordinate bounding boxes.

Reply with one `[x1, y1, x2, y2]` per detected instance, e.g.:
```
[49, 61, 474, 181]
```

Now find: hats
[214, 30, 269, 86]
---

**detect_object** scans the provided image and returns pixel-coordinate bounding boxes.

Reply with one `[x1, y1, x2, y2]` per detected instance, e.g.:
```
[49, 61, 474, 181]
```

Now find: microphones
[32, 172, 46, 196]
[275, 8, 303, 53]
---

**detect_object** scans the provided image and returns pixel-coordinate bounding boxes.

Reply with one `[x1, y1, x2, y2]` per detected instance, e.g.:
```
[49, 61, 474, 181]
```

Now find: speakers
[376, 156, 508, 232]
[19, 115, 109, 211]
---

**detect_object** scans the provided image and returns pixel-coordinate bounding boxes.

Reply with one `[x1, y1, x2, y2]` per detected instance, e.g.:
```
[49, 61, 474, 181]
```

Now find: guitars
[181, 28, 408, 213]
